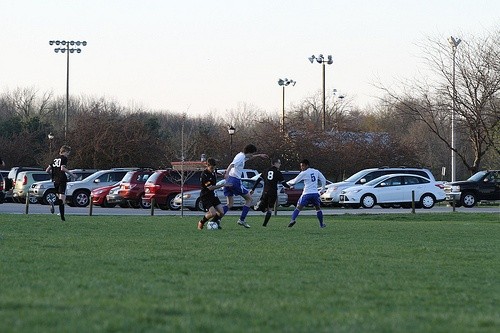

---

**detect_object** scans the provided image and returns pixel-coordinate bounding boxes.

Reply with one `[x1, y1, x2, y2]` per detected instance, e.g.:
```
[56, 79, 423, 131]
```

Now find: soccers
[206, 222, 218, 230]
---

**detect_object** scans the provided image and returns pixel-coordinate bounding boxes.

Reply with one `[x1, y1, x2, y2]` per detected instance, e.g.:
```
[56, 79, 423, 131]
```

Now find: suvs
[445, 169, 500, 208]
[118, 168, 157, 209]
[141, 168, 225, 211]
[0, 166, 138, 209]
[278, 169, 333, 208]
[214, 169, 260, 184]
[318, 166, 436, 207]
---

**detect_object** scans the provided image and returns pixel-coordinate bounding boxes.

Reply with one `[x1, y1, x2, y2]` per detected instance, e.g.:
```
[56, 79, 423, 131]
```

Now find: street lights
[48, 39, 88, 146]
[227, 125, 236, 162]
[446, 36, 463, 181]
[278, 77, 297, 164]
[307, 54, 333, 139]
[48, 132, 55, 165]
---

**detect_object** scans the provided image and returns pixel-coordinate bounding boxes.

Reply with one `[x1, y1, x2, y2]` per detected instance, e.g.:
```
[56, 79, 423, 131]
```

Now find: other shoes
[320, 223, 326, 227]
[197, 221, 203, 230]
[210, 220, 222, 229]
[288, 220, 296, 227]
[50, 202, 54, 214]
[237, 219, 250, 228]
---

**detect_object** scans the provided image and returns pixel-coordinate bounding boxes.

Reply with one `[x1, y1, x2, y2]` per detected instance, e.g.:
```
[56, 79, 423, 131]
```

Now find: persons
[207, 143, 269, 229]
[284, 158, 328, 228]
[198, 158, 224, 230]
[250, 158, 291, 225]
[45, 145, 76, 221]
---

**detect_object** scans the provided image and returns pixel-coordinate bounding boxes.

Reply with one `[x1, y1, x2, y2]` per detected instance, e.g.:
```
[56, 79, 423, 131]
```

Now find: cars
[173, 178, 289, 212]
[339, 173, 446, 209]
